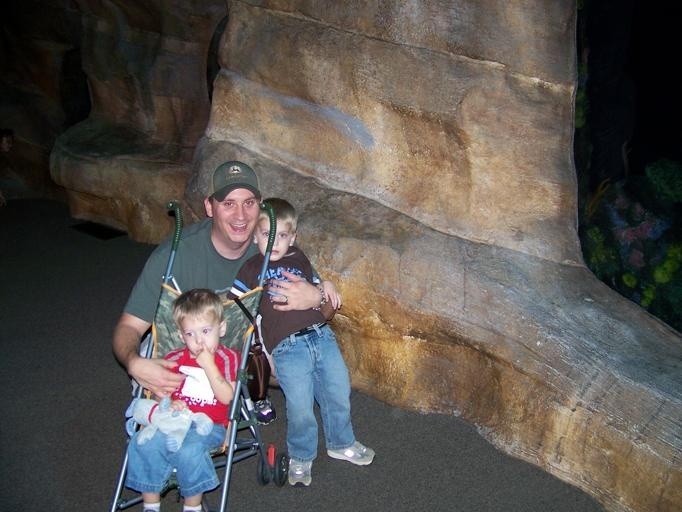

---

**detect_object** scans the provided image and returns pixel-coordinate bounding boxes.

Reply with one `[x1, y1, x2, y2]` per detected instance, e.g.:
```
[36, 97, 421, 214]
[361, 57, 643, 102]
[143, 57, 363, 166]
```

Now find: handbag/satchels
[235, 298, 271, 400]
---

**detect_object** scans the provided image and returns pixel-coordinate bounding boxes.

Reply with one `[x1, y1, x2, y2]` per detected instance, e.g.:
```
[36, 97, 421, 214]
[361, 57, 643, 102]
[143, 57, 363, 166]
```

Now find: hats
[207, 161, 260, 202]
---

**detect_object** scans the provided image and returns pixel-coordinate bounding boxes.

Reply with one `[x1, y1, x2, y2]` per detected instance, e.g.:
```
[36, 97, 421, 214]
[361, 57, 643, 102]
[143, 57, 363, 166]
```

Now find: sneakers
[288, 457, 314, 488]
[327, 439, 376, 466]
[253, 398, 277, 425]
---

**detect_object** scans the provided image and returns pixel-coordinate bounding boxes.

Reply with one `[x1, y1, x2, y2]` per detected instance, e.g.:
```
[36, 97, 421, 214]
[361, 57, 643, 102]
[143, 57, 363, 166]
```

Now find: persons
[225, 198, 375, 487]
[113, 160, 334, 424]
[124, 288, 241, 512]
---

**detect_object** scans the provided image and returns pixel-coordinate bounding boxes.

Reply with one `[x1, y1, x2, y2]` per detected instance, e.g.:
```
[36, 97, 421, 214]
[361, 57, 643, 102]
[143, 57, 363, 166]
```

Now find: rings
[282, 293, 291, 303]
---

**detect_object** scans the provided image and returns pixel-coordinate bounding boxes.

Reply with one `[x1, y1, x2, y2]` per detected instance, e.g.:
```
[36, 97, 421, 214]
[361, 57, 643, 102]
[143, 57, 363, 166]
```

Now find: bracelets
[313, 286, 326, 311]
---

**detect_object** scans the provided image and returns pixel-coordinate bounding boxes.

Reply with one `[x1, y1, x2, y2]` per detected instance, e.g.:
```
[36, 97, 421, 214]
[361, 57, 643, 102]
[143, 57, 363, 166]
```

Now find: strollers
[104, 194, 302, 512]
